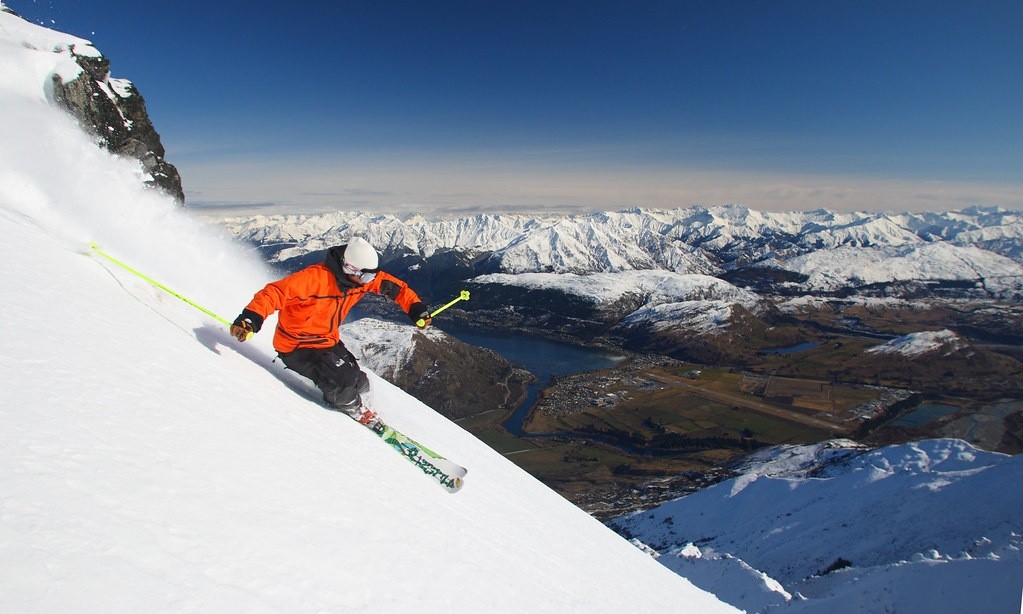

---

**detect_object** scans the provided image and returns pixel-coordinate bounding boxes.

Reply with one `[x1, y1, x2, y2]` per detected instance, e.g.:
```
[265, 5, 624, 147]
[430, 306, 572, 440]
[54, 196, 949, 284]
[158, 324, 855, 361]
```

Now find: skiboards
[332, 402, 468, 494]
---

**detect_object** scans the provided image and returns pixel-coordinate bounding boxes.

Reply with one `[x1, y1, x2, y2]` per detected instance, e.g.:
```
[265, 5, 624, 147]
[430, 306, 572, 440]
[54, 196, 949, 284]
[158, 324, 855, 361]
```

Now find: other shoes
[345, 401, 373, 424]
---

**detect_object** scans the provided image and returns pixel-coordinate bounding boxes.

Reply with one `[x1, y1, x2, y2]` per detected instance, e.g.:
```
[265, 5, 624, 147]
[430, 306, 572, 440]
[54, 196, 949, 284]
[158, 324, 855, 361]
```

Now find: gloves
[413, 309, 433, 330]
[230, 314, 261, 342]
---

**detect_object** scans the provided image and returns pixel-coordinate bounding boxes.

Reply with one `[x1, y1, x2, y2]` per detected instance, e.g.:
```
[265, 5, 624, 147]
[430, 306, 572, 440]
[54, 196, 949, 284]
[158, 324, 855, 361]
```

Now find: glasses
[358, 271, 376, 284]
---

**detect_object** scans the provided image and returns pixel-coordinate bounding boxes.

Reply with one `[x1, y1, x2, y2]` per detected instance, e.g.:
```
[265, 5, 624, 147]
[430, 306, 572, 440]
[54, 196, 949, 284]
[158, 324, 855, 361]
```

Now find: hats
[342, 237, 378, 274]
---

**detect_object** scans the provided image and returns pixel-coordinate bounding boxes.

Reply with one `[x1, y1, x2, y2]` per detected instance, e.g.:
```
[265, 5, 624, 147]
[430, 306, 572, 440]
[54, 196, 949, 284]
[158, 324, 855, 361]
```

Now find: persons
[226, 237, 435, 433]
